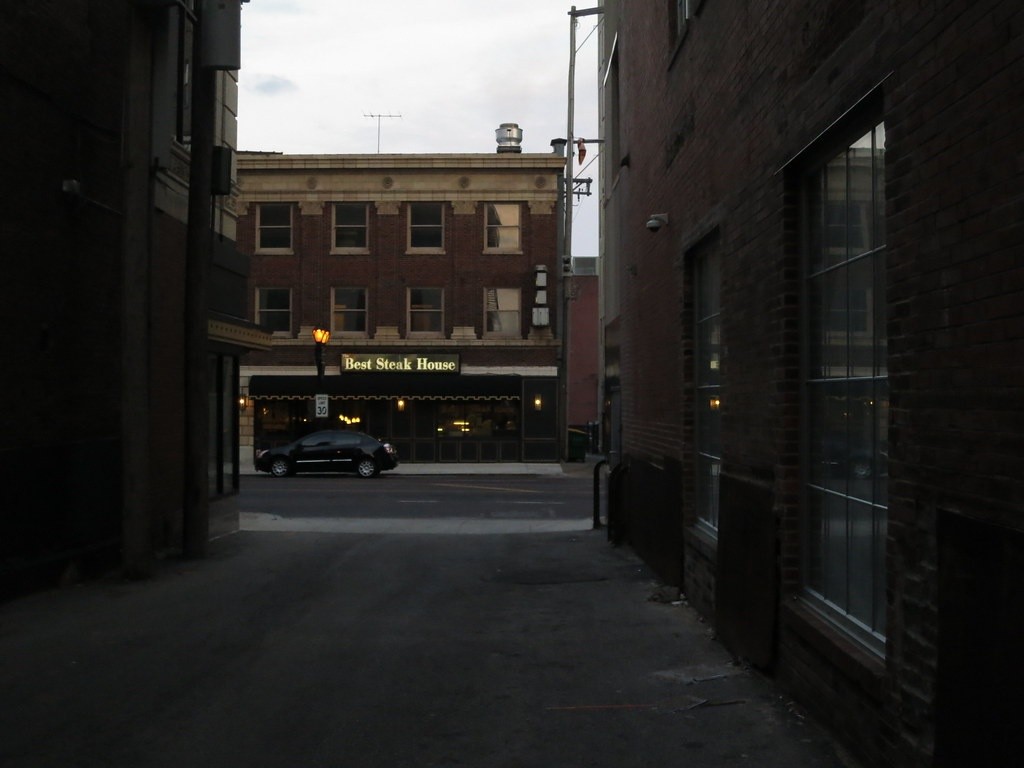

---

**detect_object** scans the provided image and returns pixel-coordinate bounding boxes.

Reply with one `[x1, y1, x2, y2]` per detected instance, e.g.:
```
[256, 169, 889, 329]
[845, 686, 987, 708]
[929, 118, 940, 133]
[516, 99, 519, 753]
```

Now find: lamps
[534, 391, 542, 415]
[239, 386, 247, 412]
[397, 398, 405, 413]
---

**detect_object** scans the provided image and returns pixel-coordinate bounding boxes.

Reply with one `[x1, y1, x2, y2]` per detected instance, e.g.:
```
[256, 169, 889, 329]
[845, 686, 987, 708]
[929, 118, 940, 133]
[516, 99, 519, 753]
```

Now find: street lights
[311, 322, 331, 426]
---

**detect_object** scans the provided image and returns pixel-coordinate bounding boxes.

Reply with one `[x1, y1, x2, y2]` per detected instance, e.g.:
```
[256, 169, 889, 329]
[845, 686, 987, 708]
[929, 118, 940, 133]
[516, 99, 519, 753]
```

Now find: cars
[253, 428, 401, 479]
